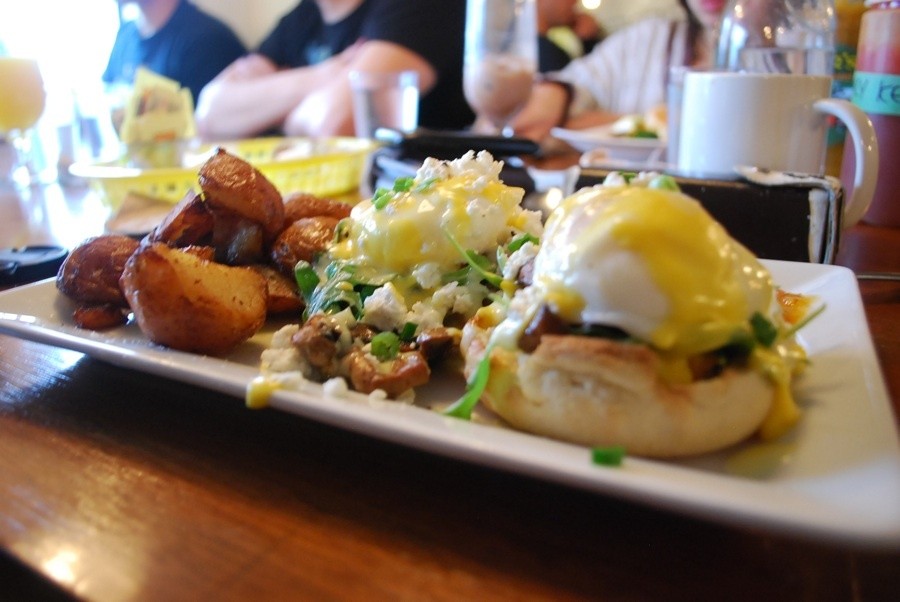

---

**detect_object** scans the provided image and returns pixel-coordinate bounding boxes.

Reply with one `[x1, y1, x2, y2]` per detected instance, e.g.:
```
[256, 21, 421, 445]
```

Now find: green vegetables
[292, 169, 638, 419]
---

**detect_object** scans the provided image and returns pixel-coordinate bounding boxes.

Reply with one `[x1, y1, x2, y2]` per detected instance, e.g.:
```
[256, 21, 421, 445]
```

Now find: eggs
[537, 184, 780, 349]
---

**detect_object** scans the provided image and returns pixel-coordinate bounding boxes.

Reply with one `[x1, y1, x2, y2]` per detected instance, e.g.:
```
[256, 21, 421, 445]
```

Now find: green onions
[595, 310, 778, 465]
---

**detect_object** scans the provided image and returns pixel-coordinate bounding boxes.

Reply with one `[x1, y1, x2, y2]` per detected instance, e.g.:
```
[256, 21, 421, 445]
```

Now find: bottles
[842, 0, 900, 226]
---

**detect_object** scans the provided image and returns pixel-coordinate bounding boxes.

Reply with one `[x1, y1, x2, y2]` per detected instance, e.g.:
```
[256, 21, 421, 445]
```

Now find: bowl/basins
[67, 130, 381, 212]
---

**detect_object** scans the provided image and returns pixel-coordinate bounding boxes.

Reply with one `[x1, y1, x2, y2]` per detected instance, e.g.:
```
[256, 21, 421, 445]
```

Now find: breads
[455, 315, 772, 460]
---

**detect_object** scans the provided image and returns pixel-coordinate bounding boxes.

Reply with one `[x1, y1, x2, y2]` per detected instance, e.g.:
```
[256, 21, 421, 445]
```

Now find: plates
[546, 124, 671, 164]
[0, 249, 900, 562]
[407, 136, 537, 167]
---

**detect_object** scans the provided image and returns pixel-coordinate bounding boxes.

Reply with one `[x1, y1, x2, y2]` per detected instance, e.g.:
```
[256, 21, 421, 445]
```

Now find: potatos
[55, 148, 353, 351]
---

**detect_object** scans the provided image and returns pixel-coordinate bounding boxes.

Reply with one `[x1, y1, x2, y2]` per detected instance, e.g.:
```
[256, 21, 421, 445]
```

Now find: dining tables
[0, 113, 900, 600]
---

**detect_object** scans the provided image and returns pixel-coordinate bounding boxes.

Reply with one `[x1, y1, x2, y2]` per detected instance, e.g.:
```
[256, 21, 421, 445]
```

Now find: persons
[103, 0, 249, 141]
[471, 0, 792, 158]
[194, 0, 476, 141]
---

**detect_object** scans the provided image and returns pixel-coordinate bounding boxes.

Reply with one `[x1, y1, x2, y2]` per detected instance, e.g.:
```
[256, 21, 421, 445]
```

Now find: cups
[680, 63, 876, 243]
[346, 65, 418, 140]
[460, 0, 540, 138]
[716, 0, 837, 88]
